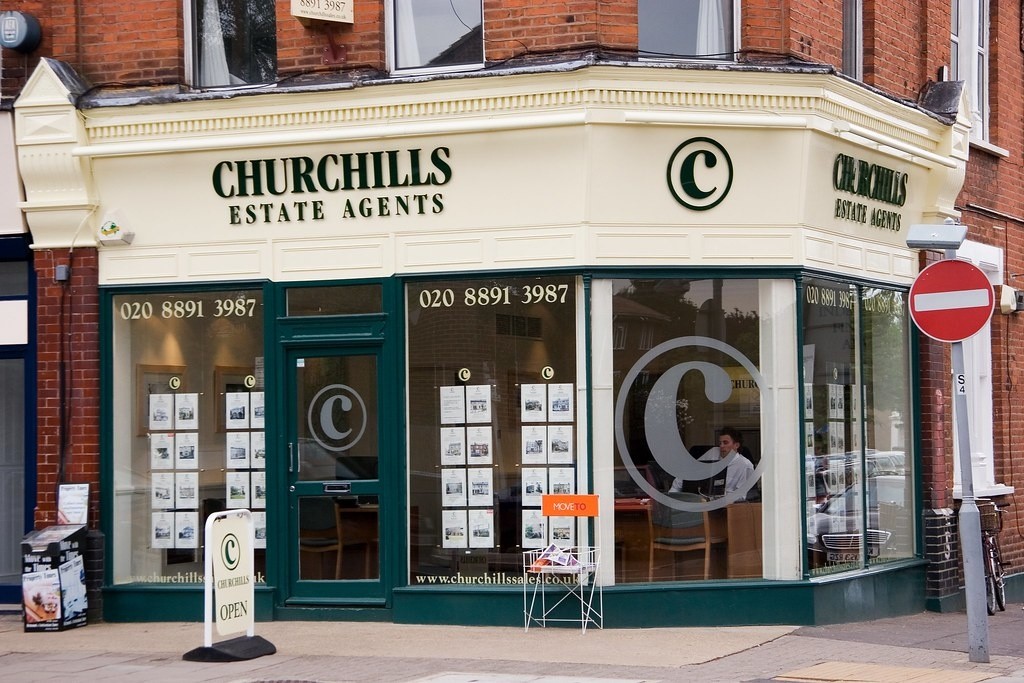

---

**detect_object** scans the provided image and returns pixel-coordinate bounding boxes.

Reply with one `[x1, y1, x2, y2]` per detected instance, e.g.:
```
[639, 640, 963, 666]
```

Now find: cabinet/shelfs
[522, 545, 604, 636]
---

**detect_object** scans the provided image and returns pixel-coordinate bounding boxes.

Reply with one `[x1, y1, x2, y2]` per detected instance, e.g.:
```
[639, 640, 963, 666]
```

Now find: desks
[614, 502, 762, 580]
[301, 506, 420, 582]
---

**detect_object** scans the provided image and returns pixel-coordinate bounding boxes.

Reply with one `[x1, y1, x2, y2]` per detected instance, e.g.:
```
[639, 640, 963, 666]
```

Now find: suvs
[806, 471, 912, 571]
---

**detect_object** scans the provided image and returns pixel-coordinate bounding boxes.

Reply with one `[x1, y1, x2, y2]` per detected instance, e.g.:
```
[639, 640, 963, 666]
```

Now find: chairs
[644, 491, 726, 582]
[298, 499, 374, 579]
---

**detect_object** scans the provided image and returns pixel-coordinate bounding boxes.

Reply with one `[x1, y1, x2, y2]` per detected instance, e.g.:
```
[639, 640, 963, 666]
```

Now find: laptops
[681, 459, 727, 498]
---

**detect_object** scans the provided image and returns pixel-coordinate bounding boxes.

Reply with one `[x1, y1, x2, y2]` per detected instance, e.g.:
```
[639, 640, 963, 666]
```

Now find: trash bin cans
[491, 484, 522, 554]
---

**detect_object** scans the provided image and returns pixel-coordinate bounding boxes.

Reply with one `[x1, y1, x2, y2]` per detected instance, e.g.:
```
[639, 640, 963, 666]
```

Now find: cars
[805, 448, 905, 500]
[297, 436, 459, 586]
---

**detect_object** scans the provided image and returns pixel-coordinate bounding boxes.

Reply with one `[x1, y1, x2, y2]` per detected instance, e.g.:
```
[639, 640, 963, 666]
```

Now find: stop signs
[908, 259, 996, 344]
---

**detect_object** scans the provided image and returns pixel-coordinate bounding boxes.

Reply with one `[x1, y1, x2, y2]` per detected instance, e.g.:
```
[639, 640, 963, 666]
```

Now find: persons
[671, 427, 755, 501]
[815, 435, 831, 503]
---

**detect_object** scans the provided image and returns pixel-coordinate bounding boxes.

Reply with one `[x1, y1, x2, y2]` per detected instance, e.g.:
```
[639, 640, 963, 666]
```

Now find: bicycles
[954, 502, 1011, 616]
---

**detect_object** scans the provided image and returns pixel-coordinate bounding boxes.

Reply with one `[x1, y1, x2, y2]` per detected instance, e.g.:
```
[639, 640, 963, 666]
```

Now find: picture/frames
[136, 363, 188, 436]
[214, 364, 259, 432]
